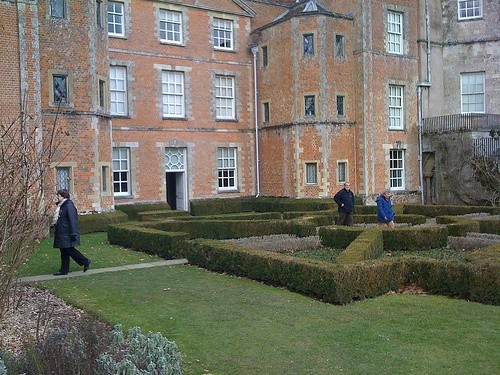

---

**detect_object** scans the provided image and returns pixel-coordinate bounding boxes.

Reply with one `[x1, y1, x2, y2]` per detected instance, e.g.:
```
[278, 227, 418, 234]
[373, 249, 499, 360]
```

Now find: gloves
[71, 235, 76, 241]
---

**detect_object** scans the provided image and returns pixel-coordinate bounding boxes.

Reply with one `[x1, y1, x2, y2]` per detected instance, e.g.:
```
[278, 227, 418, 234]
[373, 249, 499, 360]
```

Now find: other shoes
[53, 271, 69, 276]
[83, 259, 91, 272]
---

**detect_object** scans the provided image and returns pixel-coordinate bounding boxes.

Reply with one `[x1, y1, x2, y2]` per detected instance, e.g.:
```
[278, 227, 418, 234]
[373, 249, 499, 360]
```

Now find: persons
[333, 182, 355, 228]
[376, 190, 395, 228]
[50, 190, 91, 275]
